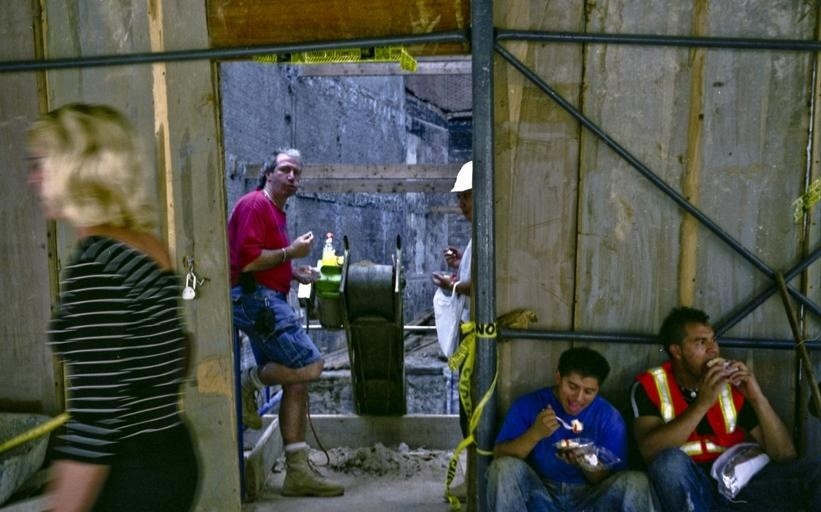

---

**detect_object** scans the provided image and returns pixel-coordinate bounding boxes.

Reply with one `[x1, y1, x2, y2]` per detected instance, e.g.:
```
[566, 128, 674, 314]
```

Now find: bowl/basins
[433, 271, 453, 283]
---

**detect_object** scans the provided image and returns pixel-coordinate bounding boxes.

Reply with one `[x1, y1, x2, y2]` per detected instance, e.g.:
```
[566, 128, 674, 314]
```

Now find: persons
[0, 101, 204, 512]
[622, 303, 800, 512]
[225, 146, 347, 500]
[431, 158, 474, 446]
[482, 345, 653, 510]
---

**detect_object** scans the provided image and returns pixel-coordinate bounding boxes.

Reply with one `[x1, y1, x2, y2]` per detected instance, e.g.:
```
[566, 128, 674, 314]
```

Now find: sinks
[0, 412, 51, 506]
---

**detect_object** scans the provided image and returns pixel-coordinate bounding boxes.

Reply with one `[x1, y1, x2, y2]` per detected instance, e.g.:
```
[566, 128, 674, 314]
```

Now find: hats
[449, 161, 473, 193]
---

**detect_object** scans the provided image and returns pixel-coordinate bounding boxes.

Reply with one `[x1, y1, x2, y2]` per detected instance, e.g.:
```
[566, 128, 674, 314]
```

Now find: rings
[542, 408, 546, 415]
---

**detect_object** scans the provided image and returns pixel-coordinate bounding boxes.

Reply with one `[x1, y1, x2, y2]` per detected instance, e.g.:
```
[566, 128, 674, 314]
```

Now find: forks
[542, 410, 574, 432]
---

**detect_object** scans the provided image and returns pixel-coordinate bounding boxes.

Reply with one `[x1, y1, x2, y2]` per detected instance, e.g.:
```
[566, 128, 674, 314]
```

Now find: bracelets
[281, 246, 288, 264]
[447, 280, 453, 290]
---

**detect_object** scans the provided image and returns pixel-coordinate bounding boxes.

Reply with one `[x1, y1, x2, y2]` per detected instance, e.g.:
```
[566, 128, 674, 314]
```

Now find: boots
[240, 370, 262, 429]
[281, 445, 344, 496]
